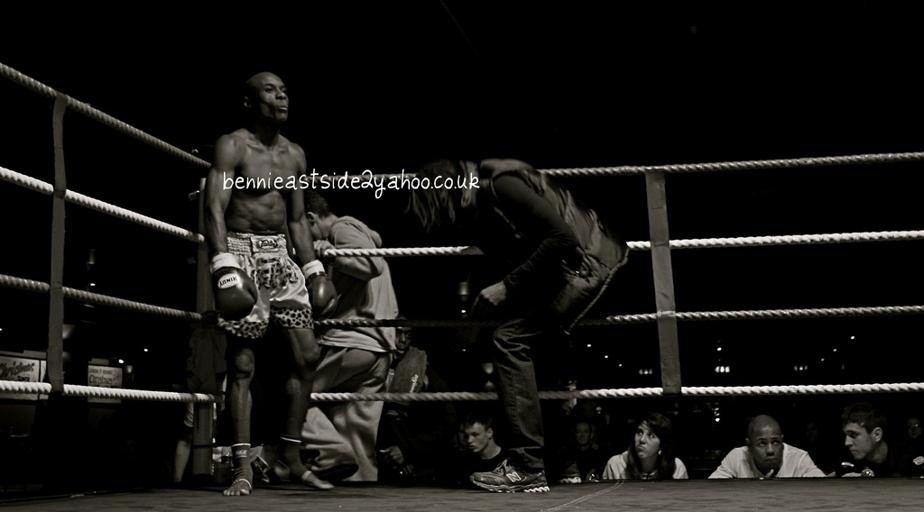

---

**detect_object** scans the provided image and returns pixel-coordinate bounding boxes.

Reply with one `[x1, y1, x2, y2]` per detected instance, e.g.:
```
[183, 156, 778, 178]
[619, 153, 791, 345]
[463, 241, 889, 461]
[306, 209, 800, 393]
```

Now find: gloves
[209, 253, 258, 321]
[301, 259, 338, 321]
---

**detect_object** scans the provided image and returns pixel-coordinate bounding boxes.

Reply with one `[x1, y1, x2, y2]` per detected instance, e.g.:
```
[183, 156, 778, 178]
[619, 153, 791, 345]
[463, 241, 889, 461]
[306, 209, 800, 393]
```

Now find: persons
[302, 185, 402, 482]
[203, 71, 340, 498]
[173, 312, 924, 486]
[408, 155, 632, 495]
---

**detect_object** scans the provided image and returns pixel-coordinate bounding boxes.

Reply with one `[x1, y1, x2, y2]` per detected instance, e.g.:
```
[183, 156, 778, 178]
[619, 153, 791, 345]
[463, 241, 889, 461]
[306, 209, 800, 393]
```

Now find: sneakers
[305, 452, 359, 481]
[469, 459, 582, 494]
